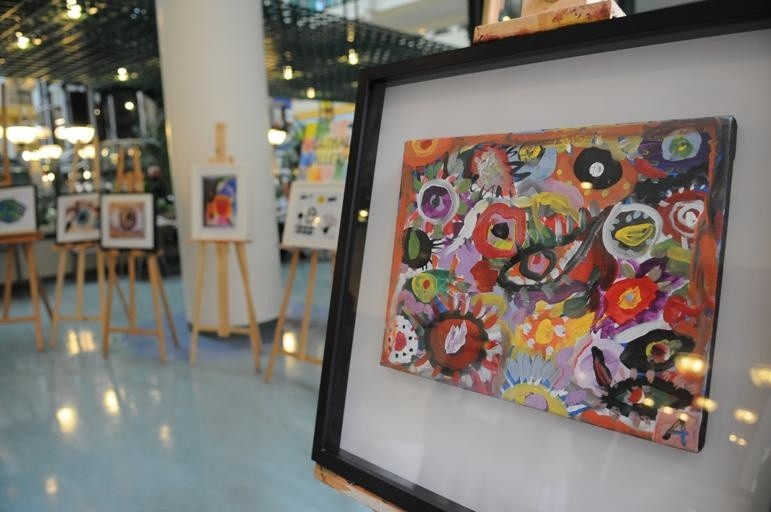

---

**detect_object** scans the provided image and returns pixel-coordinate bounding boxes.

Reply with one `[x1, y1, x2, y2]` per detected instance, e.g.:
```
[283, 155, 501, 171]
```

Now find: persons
[145, 165, 170, 212]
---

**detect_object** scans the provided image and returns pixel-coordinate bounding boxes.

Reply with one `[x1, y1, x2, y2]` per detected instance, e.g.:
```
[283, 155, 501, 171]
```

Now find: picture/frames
[191, 165, 247, 239]
[312, 0, 771, 511]
[0, 184, 157, 250]
[282, 180, 346, 251]
